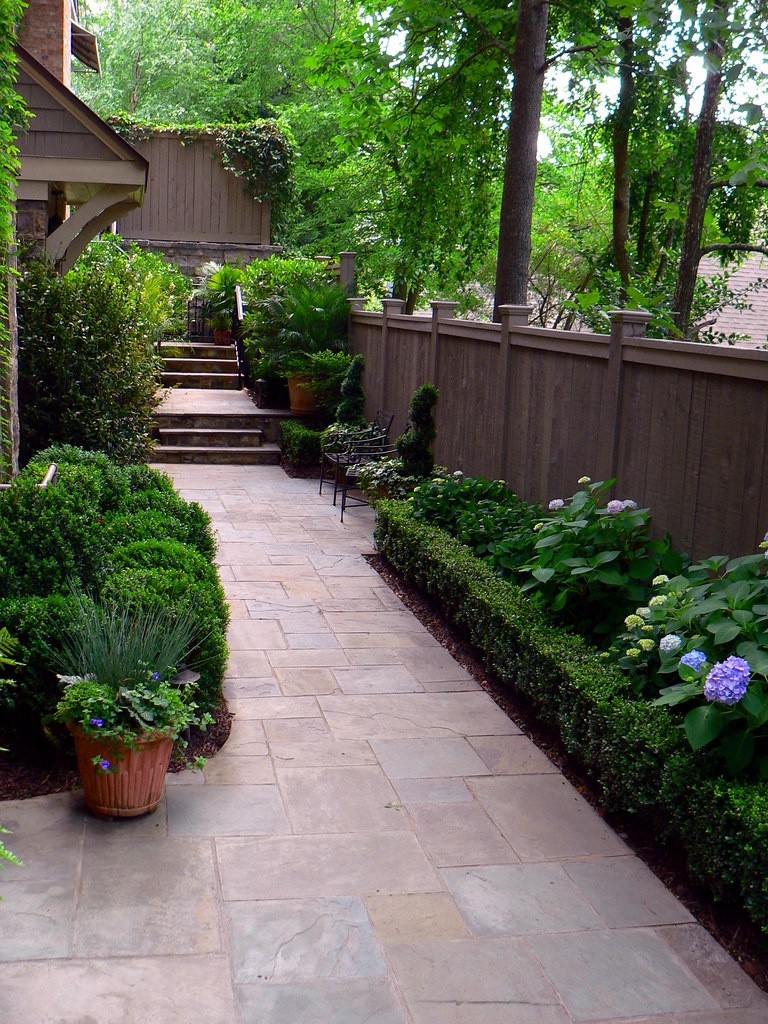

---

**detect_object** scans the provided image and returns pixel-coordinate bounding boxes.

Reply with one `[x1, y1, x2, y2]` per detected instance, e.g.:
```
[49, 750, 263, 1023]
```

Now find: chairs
[341, 424, 413, 523]
[319, 410, 395, 506]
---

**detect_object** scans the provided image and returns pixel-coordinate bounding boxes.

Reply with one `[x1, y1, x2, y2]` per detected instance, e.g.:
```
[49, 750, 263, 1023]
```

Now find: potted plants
[191, 261, 240, 345]
[246, 280, 350, 410]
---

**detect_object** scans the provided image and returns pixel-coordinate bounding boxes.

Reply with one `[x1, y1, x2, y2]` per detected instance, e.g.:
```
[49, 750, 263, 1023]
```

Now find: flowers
[40, 577, 215, 775]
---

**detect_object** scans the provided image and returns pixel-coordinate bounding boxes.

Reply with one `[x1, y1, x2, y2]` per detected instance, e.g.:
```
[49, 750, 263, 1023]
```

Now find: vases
[69, 726, 177, 820]
